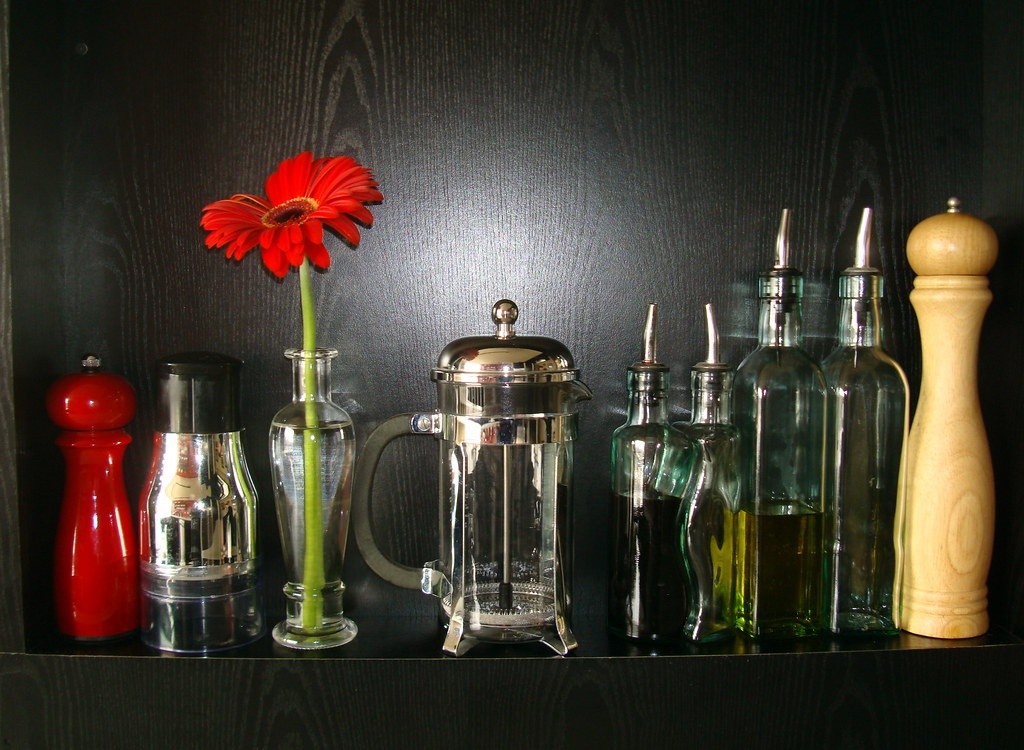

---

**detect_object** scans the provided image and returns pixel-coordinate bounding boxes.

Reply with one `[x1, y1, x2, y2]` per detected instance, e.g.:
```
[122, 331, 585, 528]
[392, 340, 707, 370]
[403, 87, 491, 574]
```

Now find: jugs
[351, 299, 594, 656]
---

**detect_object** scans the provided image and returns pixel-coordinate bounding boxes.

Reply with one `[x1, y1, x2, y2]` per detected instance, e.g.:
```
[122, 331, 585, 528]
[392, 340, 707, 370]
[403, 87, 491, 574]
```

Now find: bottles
[736, 208, 908, 645]
[893, 197, 994, 640]
[46, 355, 139, 638]
[612, 304, 760, 645]
[140, 350, 258, 654]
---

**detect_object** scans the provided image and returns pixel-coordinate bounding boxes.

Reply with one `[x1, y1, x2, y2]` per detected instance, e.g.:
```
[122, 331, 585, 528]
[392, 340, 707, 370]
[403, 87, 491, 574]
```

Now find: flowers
[200, 150, 383, 348]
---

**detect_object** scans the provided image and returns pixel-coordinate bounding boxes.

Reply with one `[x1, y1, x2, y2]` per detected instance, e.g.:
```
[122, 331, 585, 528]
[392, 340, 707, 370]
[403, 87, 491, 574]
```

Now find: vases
[269, 347, 357, 650]
